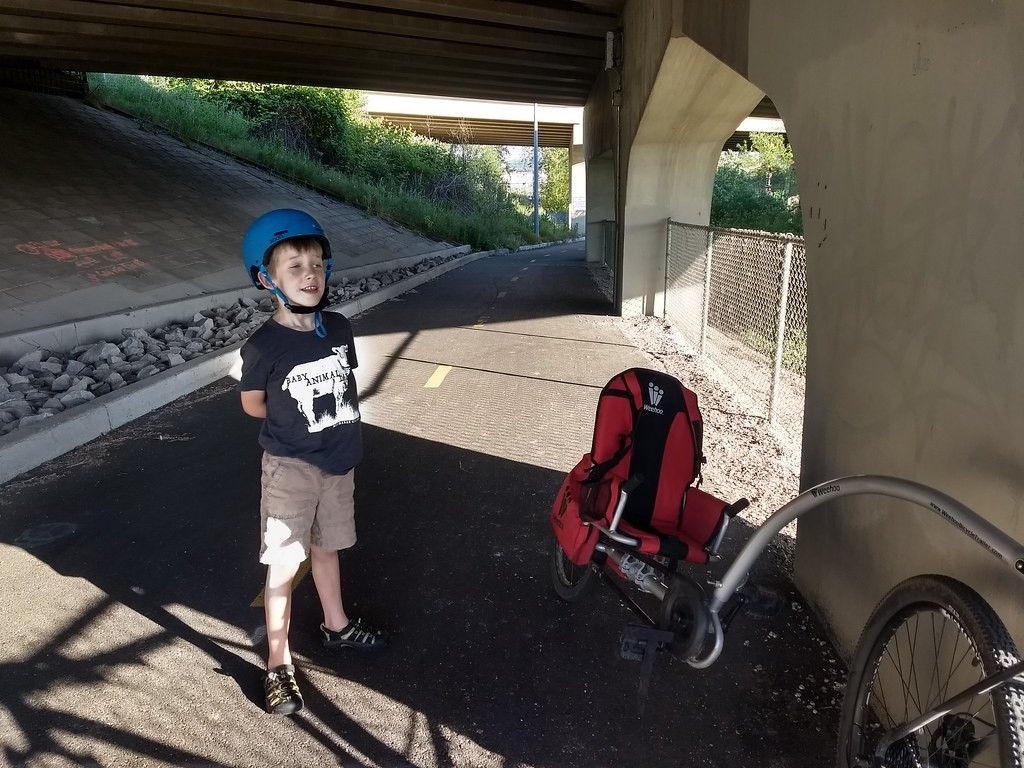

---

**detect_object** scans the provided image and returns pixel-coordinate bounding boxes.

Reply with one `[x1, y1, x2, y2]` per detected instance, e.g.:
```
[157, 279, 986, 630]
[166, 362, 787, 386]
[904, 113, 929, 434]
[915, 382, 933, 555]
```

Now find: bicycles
[836, 573, 1023, 768]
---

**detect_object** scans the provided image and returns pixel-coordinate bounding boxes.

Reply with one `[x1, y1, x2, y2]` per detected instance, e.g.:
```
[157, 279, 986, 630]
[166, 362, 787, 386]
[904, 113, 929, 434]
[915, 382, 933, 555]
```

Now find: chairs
[553, 365, 749, 659]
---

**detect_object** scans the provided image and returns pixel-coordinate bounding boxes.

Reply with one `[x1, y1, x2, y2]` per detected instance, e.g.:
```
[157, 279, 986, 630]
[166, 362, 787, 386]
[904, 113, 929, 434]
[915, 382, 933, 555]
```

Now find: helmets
[241, 208, 332, 290]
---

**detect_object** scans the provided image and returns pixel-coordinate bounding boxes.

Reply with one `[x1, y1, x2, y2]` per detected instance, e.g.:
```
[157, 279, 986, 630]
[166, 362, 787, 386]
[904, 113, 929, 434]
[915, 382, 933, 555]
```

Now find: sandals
[262, 662, 304, 715]
[321, 618, 391, 649]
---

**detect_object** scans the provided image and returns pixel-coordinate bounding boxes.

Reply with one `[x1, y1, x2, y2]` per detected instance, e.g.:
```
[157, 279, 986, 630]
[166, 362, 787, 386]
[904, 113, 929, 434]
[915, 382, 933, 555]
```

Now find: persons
[239, 209, 391, 715]
